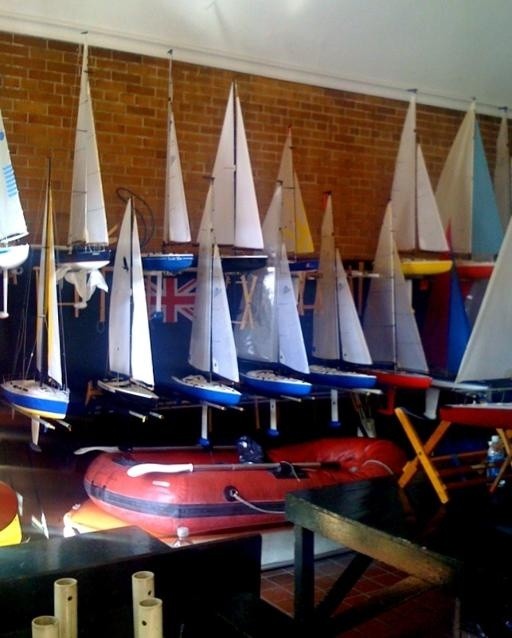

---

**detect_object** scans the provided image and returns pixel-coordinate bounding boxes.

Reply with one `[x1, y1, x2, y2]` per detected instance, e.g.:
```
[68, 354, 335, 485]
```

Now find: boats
[58, 501, 358, 582]
[68, 429, 423, 540]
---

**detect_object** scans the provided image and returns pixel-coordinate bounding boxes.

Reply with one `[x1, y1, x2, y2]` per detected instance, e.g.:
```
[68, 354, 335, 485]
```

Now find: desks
[283, 453, 512, 636]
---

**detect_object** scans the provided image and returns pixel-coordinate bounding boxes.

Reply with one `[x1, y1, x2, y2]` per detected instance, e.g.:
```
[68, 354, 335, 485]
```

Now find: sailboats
[1, 156, 71, 445]
[84, 199, 164, 424]
[29, 30, 111, 319]
[304, 191, 383, 428]
[363, 87, 511, 427]
[170, 174, 246, 448]
[1, 108, 31, 319]
[276, 125, 320, 317]
[140, 49, 194, 313]
[233, 180, 312, 437]
[187, 72, 269, 330]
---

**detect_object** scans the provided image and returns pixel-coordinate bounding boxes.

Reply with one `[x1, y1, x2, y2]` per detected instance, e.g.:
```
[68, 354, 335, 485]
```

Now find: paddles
[127, 460, 339, 480]
[75, 446, 237, 455]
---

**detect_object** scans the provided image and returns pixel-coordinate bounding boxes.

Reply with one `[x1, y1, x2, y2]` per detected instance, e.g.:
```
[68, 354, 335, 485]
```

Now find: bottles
[487, 435, 507, 486]
[170, 526, 193, 548]
